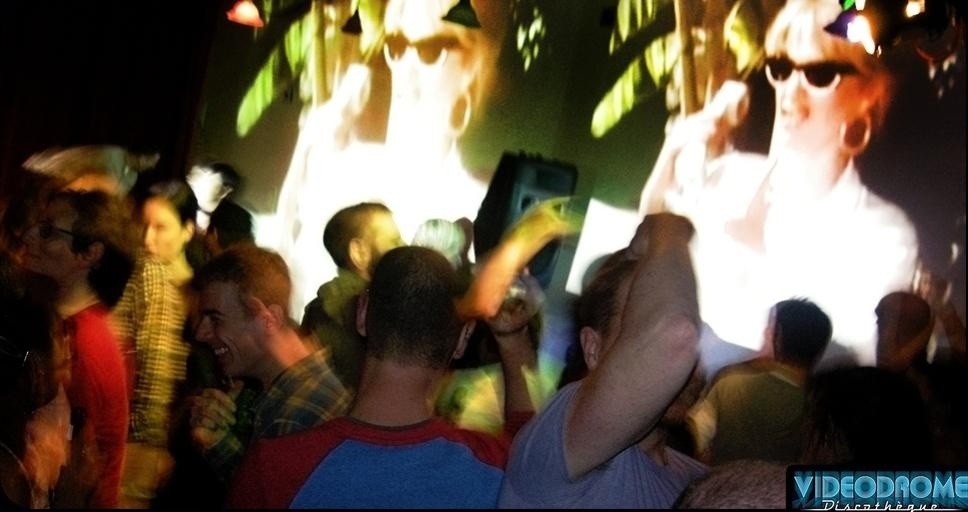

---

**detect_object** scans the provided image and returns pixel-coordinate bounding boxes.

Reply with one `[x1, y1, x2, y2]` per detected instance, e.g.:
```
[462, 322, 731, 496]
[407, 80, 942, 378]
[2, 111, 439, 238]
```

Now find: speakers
[475, 154, 596, 299]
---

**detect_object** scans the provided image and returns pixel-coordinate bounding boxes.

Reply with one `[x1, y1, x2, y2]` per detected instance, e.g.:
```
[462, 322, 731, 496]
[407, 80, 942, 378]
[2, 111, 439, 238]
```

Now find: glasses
[37, 221, 72, 239]
[384, 32, 457, 65]
[765, 56, 852, 91]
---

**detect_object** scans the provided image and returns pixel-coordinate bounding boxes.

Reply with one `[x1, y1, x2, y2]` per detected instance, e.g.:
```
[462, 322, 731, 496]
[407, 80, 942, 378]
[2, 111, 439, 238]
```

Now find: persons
[0, 0, 967, 512]
